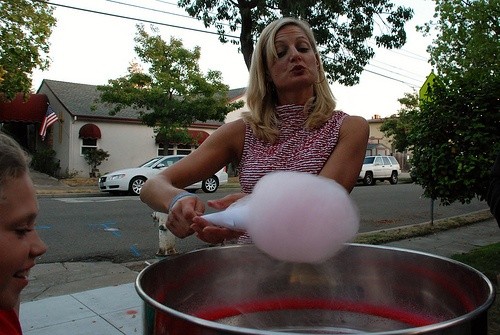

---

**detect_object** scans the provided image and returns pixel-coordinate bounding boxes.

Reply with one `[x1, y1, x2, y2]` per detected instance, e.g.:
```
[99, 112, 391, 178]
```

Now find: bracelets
[168, 191, 195, 212]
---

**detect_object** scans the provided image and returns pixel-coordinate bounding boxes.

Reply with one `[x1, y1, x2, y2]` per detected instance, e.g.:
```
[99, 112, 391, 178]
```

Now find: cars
[98, 154, 229, 194]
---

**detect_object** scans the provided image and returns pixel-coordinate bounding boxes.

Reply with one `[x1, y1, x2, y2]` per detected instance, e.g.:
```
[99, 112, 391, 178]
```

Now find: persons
[138, 18, 369, 245]
[0, 133, 47, 335]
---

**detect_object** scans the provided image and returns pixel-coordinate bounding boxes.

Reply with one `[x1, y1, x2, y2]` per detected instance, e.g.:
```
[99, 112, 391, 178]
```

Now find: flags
[40, 105, 58, 141]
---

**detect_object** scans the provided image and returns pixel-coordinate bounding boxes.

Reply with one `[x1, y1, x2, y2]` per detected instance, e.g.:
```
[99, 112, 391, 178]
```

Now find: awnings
[168, 129, 209, 146]
[79, 123, 101, 139]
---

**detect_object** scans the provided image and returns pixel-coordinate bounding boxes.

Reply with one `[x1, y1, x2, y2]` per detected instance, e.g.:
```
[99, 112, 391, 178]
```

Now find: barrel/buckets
[135, 238, 495, 335]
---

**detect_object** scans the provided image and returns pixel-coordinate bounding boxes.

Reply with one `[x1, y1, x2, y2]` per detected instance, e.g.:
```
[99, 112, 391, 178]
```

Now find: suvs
[356, 156, 402, 186]
